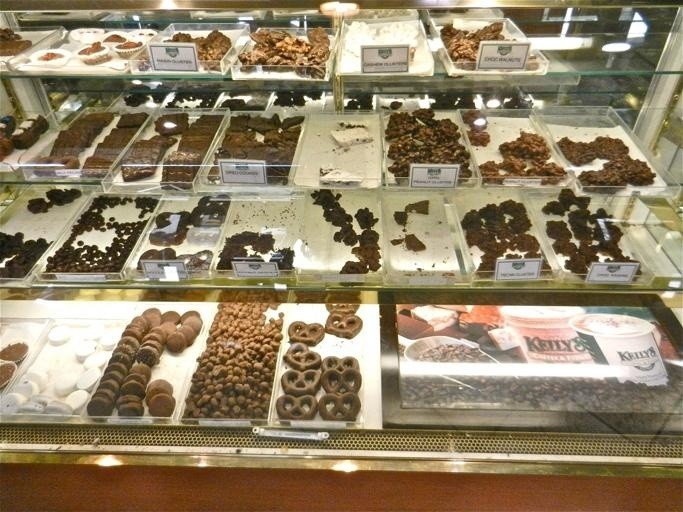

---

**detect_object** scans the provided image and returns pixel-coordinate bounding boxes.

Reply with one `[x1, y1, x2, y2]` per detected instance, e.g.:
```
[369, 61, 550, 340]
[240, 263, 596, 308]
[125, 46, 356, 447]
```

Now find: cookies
[0, 22, 655, 285]
[0, 302, 362, 421]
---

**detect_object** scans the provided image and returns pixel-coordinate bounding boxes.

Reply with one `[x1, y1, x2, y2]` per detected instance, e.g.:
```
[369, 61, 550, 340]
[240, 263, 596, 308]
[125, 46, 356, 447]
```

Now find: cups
[497, 305, 597, 363]
[566, 312, 668, 388]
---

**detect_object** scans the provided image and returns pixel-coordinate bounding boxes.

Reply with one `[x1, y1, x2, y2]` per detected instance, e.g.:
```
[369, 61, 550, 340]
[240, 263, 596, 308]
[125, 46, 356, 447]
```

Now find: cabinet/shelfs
[0, 0, 681, 511]
